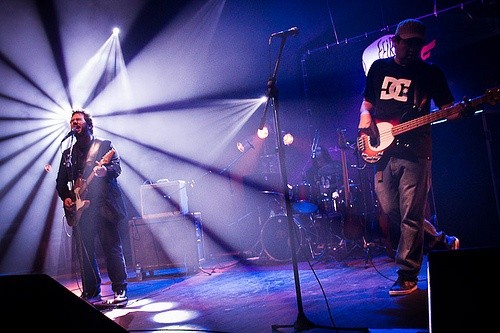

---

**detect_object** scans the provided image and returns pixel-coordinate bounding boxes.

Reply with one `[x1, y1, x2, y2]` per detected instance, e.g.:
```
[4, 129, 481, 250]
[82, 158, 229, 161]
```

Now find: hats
[394, 19, 425, 42]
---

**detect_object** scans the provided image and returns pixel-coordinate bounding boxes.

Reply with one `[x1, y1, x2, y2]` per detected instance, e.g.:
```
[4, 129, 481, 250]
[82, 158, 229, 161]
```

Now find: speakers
[0, 274, 129, 333]
[427, 247, 500, 333]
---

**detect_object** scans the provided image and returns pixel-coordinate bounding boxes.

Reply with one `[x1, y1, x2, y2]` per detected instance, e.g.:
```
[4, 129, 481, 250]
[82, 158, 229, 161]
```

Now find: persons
[356, 19, 460, 296]
[56, 109, 128, 304]
[302, 144, 346, 255]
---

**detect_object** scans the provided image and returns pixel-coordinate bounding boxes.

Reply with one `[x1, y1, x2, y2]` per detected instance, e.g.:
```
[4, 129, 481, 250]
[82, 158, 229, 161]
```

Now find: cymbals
[318, 161, 341, 176]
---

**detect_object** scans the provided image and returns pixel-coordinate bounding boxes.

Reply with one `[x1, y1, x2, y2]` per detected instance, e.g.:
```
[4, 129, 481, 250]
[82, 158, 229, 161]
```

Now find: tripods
[258, 34, 385, 333]
[219, 146, 262, 269]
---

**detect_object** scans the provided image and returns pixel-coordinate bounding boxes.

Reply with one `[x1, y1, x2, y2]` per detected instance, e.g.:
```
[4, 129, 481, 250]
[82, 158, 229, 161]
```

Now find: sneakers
[113, 287, 128, 309]
[388, 276, 417, 295]
[80, 293, 102, 303]
[444, 235, 460, 251]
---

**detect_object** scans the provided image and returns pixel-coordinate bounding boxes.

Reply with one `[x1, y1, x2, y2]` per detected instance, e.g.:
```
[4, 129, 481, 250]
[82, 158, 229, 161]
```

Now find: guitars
[62, 147, 115, 227]
[357, 88, 500, 164]
[260, 213, 319, 263]
[335, 126, 366, 240]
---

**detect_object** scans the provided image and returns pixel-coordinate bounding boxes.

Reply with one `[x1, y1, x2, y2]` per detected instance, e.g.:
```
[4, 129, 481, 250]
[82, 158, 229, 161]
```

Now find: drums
[311, 211, 344, 243]
[319, 193, 343, 217]
[254, 191, 284, 214]
[289, 183, 321, 214]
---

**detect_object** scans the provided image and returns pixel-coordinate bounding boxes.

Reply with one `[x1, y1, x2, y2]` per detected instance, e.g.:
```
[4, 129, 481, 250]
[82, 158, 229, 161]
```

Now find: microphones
[247, 140, 255, 149]
[272, 27, 298, 38]
[62, 129, 76, 142]
